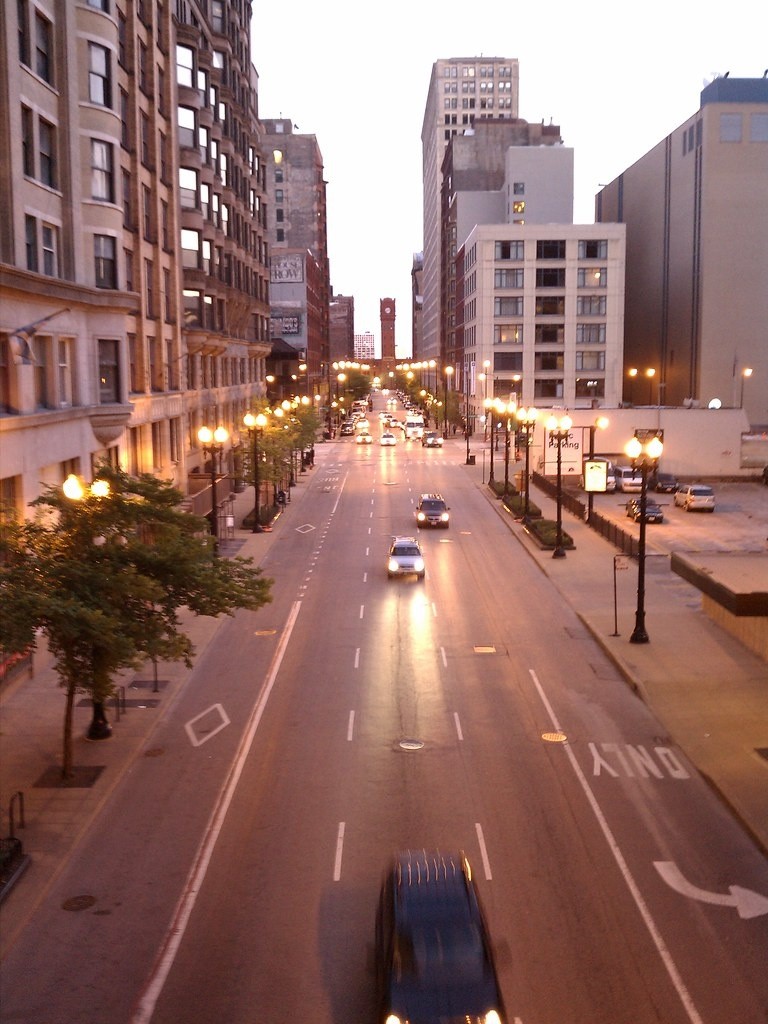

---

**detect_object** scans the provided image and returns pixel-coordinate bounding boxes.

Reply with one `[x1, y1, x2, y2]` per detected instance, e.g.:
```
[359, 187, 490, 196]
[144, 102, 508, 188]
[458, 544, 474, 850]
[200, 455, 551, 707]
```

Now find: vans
[673, 484, 716, 512]
[580, 458, 648, 493]
[405, 416, 424, 439]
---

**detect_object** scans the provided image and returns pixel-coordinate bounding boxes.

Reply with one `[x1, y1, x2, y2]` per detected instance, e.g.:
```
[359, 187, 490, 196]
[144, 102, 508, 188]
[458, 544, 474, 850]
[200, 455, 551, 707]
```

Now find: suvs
[421, 431, 443, 447]
[415, 493, 450, 529]
[388, 536, 425, 579]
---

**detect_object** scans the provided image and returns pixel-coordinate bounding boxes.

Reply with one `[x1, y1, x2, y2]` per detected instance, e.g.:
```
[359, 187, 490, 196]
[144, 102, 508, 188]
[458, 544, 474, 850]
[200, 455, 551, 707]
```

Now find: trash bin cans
[443, 432, 446, 439]
[324, 433, 329, 439]
[310, 450, 314, 457]
[470, 455, 476, 465]
[278, 490, 287, 505]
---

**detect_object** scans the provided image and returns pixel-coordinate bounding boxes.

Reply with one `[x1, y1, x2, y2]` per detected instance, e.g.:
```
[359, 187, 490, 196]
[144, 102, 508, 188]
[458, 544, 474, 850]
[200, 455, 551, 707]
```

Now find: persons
[323, 426, 330, 439]
[310, 447, 315, 466]
[453, 424, 457, 435]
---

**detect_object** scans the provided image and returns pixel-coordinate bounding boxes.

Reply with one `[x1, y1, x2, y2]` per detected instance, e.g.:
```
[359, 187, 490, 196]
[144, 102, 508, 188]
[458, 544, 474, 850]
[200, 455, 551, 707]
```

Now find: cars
[625, 497, 664, 523]
[647, 474, 679, 493]
[378, 411, 406, 446]
[396, 389, 422, 416]
[387, 396, 397, 404]
[375, 850, 508, 1024]
[339, 393, 373, 444]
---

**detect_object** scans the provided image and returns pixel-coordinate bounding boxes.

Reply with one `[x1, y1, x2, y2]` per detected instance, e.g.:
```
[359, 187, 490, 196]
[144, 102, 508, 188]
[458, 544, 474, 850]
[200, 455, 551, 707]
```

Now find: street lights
[479, 359, 538, 525]
[198, 426, 230, 567]
[62, 472, 114, 740]
[243, 396, 309, 534]
[444, 365, 454, 440]
[624, 437, 664, 644]
[544, 415, 573, 559]
[414, 360, 438, 431]
